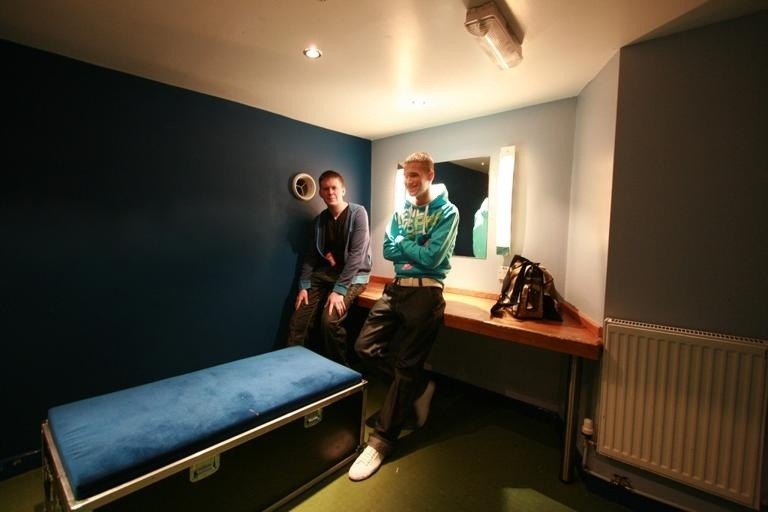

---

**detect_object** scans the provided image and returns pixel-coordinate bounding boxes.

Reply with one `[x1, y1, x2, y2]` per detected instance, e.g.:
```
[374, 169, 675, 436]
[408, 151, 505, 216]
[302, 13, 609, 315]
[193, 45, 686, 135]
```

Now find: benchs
[41, 346, 370, 512]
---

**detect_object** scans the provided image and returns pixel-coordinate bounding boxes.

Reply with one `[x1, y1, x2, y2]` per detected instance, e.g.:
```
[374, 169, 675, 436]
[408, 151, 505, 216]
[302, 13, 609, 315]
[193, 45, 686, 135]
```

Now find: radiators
[584, 317, 768, 512]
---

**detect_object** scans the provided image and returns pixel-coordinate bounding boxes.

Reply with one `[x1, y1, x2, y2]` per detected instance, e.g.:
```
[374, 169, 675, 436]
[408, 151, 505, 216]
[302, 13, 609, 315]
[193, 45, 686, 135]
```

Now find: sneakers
[414, 381, 435, 427]
[349, 445, 384, 481]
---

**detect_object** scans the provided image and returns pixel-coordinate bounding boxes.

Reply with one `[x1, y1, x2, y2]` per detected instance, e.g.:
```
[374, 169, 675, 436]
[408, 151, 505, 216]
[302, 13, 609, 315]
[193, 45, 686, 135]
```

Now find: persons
[347, 151, 461, 481]
[472, 195, 488, 257]
[287, 170, 373, 367]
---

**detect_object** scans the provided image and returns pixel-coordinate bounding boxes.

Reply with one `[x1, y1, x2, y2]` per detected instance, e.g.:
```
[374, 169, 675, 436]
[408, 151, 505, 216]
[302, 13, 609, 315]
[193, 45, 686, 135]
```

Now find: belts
[392, 277, 443, 290]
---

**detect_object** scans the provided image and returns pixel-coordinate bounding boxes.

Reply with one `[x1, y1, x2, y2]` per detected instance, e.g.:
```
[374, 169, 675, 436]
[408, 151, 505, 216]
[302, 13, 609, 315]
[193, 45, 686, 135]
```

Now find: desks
[355, 281, 602, 484]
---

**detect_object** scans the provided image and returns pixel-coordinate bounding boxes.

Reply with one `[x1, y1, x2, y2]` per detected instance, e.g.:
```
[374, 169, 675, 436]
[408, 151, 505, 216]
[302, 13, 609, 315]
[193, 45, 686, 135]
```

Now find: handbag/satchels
[491, 254, 562, 323]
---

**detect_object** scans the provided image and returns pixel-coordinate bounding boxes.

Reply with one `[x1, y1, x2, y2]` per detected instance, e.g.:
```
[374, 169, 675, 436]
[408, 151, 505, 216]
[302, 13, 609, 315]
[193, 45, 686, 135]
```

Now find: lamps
[464, 1, 523, 70]
